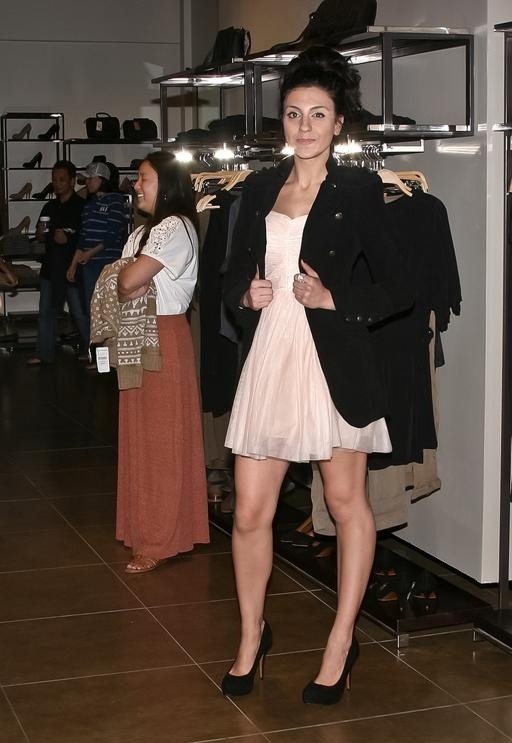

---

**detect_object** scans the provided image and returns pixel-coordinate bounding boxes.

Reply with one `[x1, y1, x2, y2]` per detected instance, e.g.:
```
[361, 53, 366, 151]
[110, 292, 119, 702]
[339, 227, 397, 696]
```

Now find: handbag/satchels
[210, 26, 251, 64]
[83, 112, 121, 138]
[123, 118, 158, 140]
[298, 0, 377, 45]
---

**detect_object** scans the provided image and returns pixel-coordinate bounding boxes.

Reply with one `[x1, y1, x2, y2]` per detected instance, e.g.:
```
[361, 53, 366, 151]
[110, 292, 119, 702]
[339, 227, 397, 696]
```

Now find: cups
[39, 216, 50, 232]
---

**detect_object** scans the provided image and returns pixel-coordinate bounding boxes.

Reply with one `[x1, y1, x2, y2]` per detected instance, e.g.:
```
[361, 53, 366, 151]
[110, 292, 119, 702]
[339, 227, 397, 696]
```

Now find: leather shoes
[207, 486, 222, 513]
[280, 513, 336, 558]
[369, 569, 438, 618]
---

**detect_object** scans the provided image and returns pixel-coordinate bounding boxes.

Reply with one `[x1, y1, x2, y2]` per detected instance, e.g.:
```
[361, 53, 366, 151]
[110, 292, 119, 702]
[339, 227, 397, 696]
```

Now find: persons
[112, 150, 210, 575]
[24, 159, 91, 365]
[64, 159, 126, 371]
[212, 46, 395, 704]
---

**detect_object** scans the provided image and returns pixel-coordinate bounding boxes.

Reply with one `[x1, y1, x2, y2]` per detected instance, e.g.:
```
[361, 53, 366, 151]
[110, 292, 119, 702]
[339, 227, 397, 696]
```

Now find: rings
[297, 272, 305, 282]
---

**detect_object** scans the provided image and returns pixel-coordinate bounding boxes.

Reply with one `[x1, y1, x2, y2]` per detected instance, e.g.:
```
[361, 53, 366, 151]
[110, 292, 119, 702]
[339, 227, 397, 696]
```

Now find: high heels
[32, 182, 54, 199]
[23, 152, 42, 167]
[222, 619, 273, 695]
[38, 123, 60, 140]
[12, 123, 32, 140]
[10, 183, 32, 199]
[10, 216, 31, 233]
[302, 636, 359, 705]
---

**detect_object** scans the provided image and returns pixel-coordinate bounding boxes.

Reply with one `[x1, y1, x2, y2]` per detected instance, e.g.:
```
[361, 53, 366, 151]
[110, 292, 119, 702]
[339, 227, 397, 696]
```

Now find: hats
[81, 163, 111, 180]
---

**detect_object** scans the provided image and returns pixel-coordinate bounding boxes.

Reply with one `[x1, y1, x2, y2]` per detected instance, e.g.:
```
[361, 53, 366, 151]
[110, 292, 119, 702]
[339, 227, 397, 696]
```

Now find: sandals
[124, 554, 171, 574]
